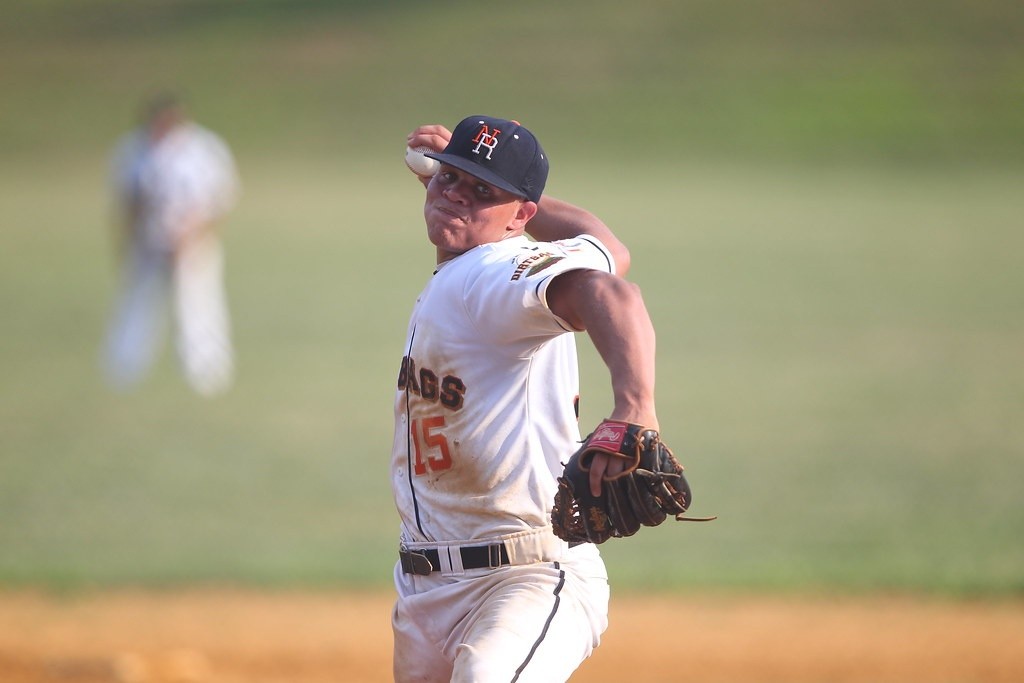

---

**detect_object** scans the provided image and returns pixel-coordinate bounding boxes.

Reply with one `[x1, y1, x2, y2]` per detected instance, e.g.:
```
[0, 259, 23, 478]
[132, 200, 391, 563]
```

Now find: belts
[399, 541, 509, 577]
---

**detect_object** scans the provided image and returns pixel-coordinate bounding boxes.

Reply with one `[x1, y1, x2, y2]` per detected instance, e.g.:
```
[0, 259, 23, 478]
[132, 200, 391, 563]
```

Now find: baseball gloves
[550, 418, 719, 551]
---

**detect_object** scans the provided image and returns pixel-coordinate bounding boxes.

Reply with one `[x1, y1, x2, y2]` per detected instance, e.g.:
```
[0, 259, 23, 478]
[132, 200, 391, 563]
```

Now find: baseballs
[405, 143, 442, 179]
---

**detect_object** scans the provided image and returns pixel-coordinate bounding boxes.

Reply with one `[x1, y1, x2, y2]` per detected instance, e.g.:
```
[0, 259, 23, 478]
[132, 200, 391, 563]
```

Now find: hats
[424, 115, 550, 204]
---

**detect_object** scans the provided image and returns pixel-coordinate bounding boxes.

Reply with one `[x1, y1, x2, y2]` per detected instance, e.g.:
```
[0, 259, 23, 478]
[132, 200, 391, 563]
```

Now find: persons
[108, 97, 234, 386]
[392, 116, 691, 683]
